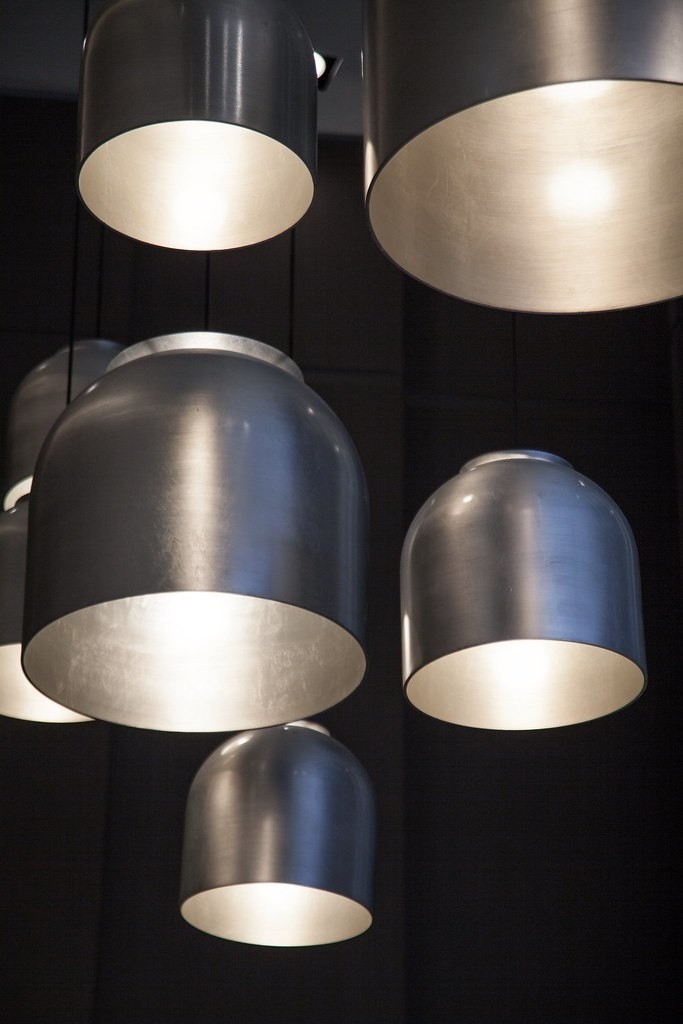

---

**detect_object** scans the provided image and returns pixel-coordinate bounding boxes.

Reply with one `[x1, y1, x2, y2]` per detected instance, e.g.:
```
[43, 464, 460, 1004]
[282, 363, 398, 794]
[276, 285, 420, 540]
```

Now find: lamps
[397, 320, 645, 733]
[180, 231, 376, 950]
[358, 0, 683, 315]
[0, 223, 131, 509]
[78, 0, 320, 251]
[26, 252, 369, 732]
[0, 0, 96, 724]
